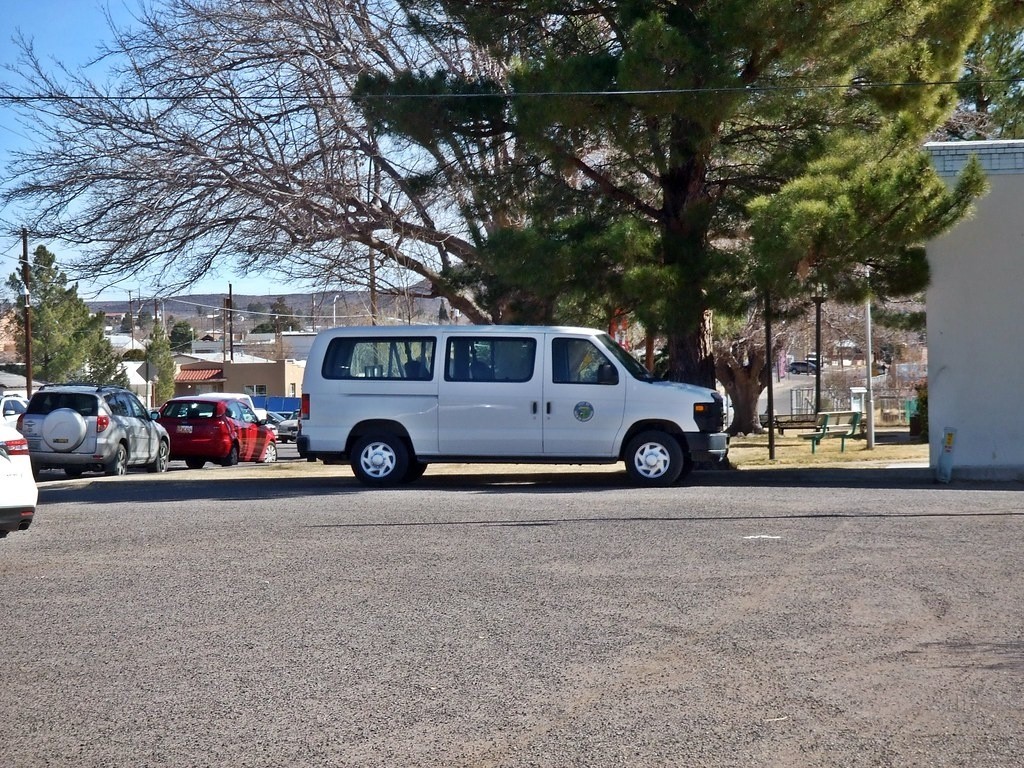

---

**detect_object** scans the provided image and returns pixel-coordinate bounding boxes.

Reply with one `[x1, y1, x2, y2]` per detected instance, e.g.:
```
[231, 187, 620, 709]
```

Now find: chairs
[188, 407, 201, 418]
[404, 360, 428, 378]
[471, 361, 494, 381]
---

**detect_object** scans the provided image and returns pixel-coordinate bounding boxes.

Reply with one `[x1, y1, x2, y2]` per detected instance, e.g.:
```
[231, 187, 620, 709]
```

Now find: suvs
[16, 382, 171, 477]
[0, 393, 29, 429]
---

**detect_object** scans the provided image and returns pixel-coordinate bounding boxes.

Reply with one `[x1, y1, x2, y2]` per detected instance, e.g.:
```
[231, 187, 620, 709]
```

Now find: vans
[199, 392, 254, 418]
[296, 324, 731, 489]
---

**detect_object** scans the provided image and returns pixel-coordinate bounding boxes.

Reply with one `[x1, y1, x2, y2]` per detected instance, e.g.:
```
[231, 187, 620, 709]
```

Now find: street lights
[811, 295, 830, 431]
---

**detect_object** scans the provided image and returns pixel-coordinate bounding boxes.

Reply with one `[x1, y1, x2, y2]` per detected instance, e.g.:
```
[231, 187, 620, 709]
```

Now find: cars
[266, 410, 299, 442]
[0, 415, 39, 538]
[282, 408, 300, 444]
[265, 423, 278, 439]
[789, 361, 816, 375]
[155, 396, 278, 469]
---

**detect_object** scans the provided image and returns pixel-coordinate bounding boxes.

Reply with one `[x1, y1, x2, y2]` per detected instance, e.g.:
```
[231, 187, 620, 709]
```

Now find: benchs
[759, 411, 861, 454]
